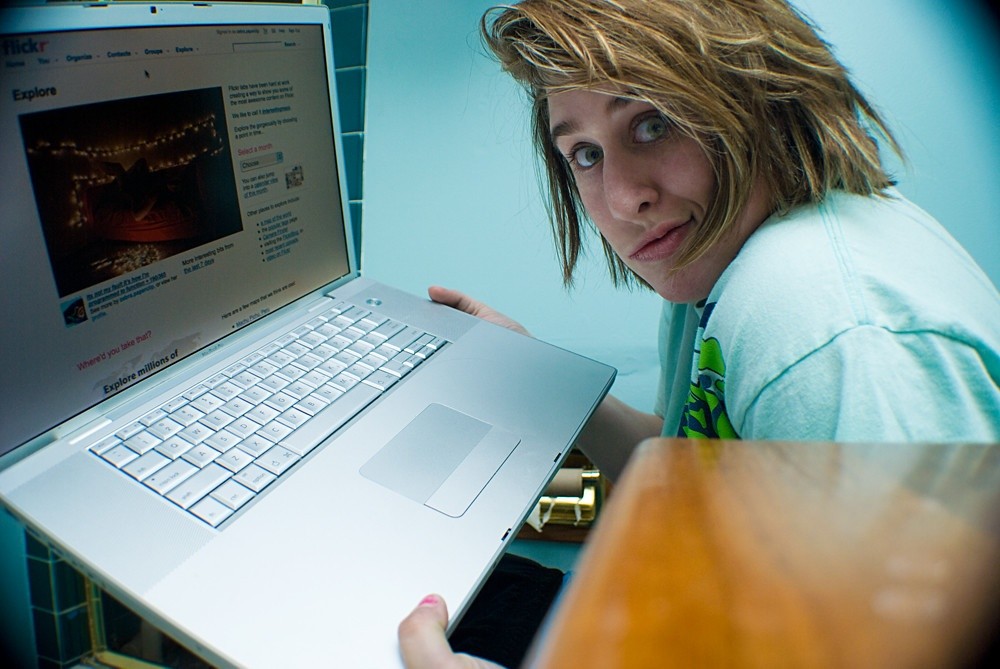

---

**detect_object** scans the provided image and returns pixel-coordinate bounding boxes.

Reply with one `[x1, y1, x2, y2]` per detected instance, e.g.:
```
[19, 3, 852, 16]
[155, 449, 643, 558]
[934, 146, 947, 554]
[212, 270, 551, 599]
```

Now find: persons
[396, 0, 1000, 669]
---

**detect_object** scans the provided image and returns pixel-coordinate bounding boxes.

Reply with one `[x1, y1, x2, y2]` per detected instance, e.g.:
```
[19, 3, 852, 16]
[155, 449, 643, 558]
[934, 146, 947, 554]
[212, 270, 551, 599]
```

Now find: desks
[519, 438, 1000, 669]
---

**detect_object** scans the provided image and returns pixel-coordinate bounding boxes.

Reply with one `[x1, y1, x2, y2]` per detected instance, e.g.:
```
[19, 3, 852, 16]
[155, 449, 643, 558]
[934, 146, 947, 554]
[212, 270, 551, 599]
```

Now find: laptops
[1, 0, 617, 669]
